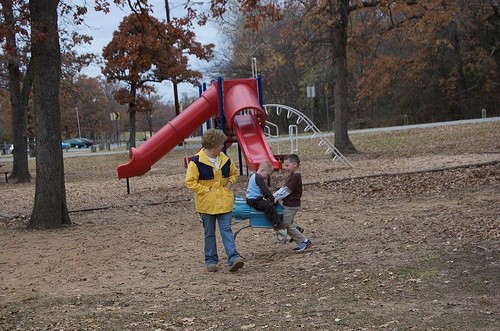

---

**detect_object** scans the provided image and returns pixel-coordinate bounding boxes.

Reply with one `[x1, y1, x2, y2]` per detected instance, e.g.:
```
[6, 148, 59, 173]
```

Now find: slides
[233, 111, 282, 171]
[117, 86, 217, 179]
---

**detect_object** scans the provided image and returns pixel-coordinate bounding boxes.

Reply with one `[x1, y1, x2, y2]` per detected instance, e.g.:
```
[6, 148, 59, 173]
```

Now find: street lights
[75, 107, 81, 138]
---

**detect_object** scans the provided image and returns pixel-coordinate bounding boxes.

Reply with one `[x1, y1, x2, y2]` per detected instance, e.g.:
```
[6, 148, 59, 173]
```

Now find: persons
[184, 128, 244, 271]
[273, 154, 314, 253]
[245, 160, 288, 231]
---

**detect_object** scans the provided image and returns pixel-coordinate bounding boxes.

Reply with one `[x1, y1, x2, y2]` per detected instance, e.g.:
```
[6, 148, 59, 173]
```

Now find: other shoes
[294, 239, 312, 252]
[229, 258, 244, 272]
[207, 263, 218, 272]
[289, 226, 304, 242]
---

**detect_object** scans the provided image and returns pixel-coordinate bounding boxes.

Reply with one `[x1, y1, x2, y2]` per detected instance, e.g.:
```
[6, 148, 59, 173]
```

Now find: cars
[79, 138, 93, 148]
[64, 139, 85, 148]
[62, 142, 71, 149]
